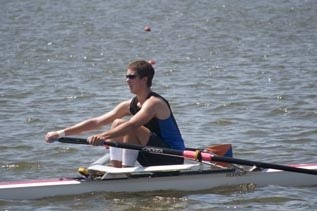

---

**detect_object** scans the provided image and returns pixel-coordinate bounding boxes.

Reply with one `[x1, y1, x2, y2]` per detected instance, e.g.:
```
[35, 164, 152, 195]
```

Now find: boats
[0, 144, 317, 201]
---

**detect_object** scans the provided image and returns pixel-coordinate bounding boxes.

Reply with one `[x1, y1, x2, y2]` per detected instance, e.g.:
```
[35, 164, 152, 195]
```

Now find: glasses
[125, 75, 140, 79]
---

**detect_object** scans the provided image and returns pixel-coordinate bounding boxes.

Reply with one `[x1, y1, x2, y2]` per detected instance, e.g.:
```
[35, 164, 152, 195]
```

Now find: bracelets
[57, 129, 66, 139]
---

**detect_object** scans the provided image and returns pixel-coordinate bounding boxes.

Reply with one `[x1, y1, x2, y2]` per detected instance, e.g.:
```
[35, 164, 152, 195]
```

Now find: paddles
[55, 136, 317, 176]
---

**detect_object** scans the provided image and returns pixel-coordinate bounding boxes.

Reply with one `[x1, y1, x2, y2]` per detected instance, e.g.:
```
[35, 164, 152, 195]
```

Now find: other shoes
[77, 168, 102, 178]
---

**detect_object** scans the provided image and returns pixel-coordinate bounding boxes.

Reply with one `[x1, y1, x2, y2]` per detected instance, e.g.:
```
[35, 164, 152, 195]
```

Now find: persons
[45, 61, 186, 177]
